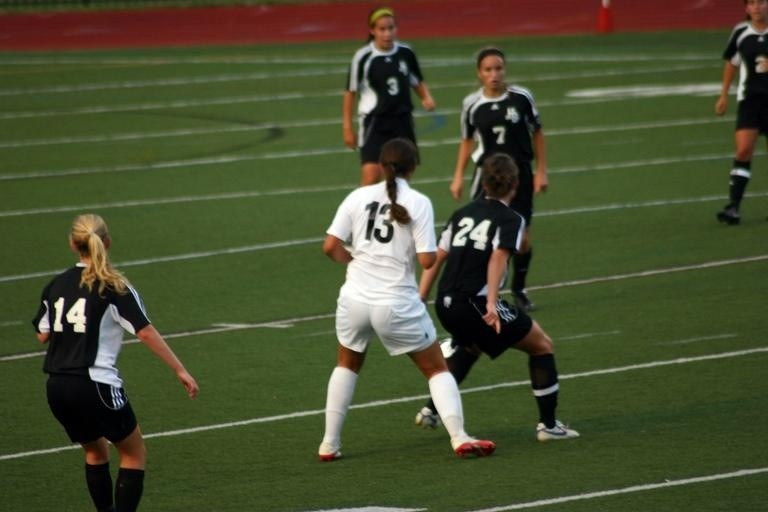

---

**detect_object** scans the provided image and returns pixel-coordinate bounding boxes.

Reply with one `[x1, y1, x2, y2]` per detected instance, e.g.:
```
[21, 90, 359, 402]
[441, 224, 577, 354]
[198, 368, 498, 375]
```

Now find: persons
[317, 139, 496, 460]
[715, 0, 768, 224]
[32, 213, 200, 512]
[414, 154, 580, 442]
[343, 6, 436, 188]
[450, 48, 549, 312]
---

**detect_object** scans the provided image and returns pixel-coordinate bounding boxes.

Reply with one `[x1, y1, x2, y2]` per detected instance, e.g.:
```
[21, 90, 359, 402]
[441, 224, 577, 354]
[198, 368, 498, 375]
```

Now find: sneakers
[536, 419, 580, 442]
[511, 289, 535, 313]
[450, 436, 498, 459]
[415, 406, 441, 430]
[717, 204, 741, 225]
[318, 442, 342, 461]
